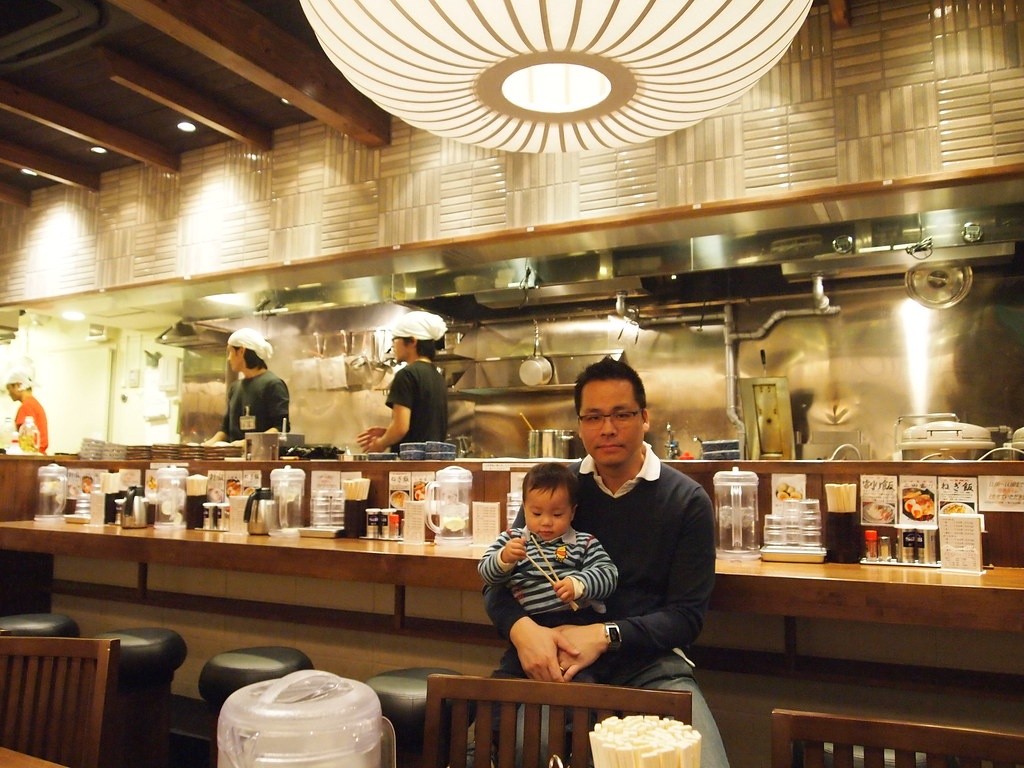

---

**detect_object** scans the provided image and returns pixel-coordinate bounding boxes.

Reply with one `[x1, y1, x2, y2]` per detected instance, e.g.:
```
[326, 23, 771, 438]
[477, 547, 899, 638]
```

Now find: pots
[517, 327, 553, 386]
[528, 429, 576, 459]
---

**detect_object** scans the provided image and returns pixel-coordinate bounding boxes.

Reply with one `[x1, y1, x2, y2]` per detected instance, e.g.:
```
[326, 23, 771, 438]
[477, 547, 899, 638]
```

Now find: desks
[0, 520, 1024, 634]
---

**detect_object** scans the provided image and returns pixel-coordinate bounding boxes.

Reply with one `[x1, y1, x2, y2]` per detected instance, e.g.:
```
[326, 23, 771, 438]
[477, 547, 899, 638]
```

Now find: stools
[793, 740, 960, 768]
[365, 667, 476, 768]
[0, 612, 85, 768]
[199, 646, 315, 768]
[93, 627, 187, 768]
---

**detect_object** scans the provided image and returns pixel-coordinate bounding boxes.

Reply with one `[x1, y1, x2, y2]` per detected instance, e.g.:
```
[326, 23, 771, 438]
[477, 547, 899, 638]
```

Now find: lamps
[299, 0, 810, 155]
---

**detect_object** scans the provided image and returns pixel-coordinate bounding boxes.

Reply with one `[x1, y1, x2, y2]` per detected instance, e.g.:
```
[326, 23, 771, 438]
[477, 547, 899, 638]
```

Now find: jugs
[424, 465, 473, 547]
[33, 463, 67, 522]
[154, 465, 189, 530]
[216, 669, 397, 768]
[269, 465, 306, 536]
[713, 466, 761, 562]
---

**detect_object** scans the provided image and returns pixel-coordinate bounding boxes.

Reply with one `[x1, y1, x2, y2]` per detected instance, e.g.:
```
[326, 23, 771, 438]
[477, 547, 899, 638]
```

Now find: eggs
[904, 499, 923, 518]
[414, 489, 425, 500]
[776, 491, 802, 500]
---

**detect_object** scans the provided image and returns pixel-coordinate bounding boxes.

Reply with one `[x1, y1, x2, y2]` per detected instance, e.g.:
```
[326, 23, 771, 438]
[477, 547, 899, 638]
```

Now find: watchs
[604, 621, 621, 652]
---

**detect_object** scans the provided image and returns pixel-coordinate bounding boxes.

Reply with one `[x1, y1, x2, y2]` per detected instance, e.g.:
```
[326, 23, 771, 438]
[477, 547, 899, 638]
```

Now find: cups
[505, 492, 522, 529]
[895, 526, 939, 564]
[104, 493, 123, 525]
[311, 489, 344, 530]
[381, 509, 396, 538]
[365, 509, 380, 538]
[187, 495, 229, 530]
[74, 484, 100, 516]
[764, 498, 823, 552]
[344, 499, 368, 538]
[826, 511, 861, 563]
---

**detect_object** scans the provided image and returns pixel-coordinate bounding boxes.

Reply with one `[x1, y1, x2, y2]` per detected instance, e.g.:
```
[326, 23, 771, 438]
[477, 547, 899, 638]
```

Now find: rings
[560, 667, 564, 672]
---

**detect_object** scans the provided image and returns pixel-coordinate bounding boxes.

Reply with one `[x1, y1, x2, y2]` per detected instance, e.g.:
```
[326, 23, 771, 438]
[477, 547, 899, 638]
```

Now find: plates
[940, 502, 975, 514]
[391, 491, 409, 508]
[79, 437, 244, 460]
[208, 479, 255, 502]
[863, 502, 895, 524]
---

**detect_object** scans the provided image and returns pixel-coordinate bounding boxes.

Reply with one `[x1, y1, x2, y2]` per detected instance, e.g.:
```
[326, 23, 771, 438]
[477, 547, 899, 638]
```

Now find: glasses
[391, 337, 400, 343]
[577, 410, 640, 424]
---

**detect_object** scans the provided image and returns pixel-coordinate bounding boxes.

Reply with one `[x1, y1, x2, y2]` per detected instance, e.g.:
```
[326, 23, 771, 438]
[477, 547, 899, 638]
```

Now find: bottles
[0, 415, 41, 455]
[388, 508, 405, 539]
[865, 530, 891, 562]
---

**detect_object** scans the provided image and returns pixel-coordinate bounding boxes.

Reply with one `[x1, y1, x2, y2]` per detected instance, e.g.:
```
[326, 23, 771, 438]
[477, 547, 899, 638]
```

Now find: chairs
[423, 674, 692, 768]
[770, 704, 1024, 768]
[0, 636, 122, 768]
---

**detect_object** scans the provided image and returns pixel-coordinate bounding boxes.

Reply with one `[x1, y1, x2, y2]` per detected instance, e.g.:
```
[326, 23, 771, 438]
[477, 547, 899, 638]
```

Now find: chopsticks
[526, 535, 579, 611]
[342, 478, 371, 499]
[588, 714, 703, 768]
[825, 483, 857, 512]
[186, 476, 208, 495]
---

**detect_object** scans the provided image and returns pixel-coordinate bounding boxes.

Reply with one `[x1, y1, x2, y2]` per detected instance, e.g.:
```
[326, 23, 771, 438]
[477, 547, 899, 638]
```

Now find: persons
[478, 462, 617, 768]
[356, 311, 448, 456]
[482, 356, 730, 768]
[7, 371, 49, 453]
[203, 327, 290, 448]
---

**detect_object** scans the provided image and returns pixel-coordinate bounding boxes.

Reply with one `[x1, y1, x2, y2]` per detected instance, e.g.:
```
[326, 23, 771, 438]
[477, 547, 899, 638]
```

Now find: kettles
[121, 484, 148, 529]
[244, 487, 271, 535]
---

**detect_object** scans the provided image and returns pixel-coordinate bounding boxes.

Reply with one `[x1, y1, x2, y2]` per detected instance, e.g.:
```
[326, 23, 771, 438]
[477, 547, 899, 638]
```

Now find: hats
[6, 373, 32, 391]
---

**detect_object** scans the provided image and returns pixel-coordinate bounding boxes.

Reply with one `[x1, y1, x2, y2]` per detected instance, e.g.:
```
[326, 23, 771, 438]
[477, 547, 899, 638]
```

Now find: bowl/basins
[903, 488, 935, 522]
[336, 454, 367, 461]
[368, 453, 398, 461]
[701, 439, 740, 460]
[399, 441, 456, 461]
